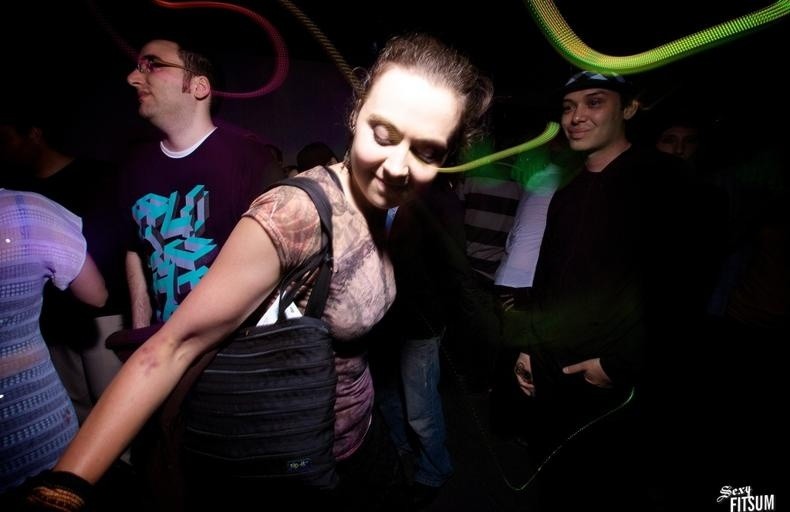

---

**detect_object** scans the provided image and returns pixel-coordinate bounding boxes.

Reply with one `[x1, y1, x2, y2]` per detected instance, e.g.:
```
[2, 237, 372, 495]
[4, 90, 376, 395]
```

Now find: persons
[2, 112, 110, 424]
[122, 36, 289, 329]
[19, 33, 498, 506]
[265, 110, 702, 307]
[511, 70, 685, 512]
[0, 184, 111, 489]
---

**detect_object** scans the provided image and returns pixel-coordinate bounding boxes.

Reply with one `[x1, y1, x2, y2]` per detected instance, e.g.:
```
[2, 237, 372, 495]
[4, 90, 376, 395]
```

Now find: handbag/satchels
[177, 316, 337, 491]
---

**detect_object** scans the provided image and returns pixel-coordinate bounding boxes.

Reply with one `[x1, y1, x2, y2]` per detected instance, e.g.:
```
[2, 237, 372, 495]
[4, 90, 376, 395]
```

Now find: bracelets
[11, 471, 97, 512]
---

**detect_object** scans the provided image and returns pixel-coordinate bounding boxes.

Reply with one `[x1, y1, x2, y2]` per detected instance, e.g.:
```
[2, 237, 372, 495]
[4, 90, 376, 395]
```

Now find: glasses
[136, 58, 197, 76]
